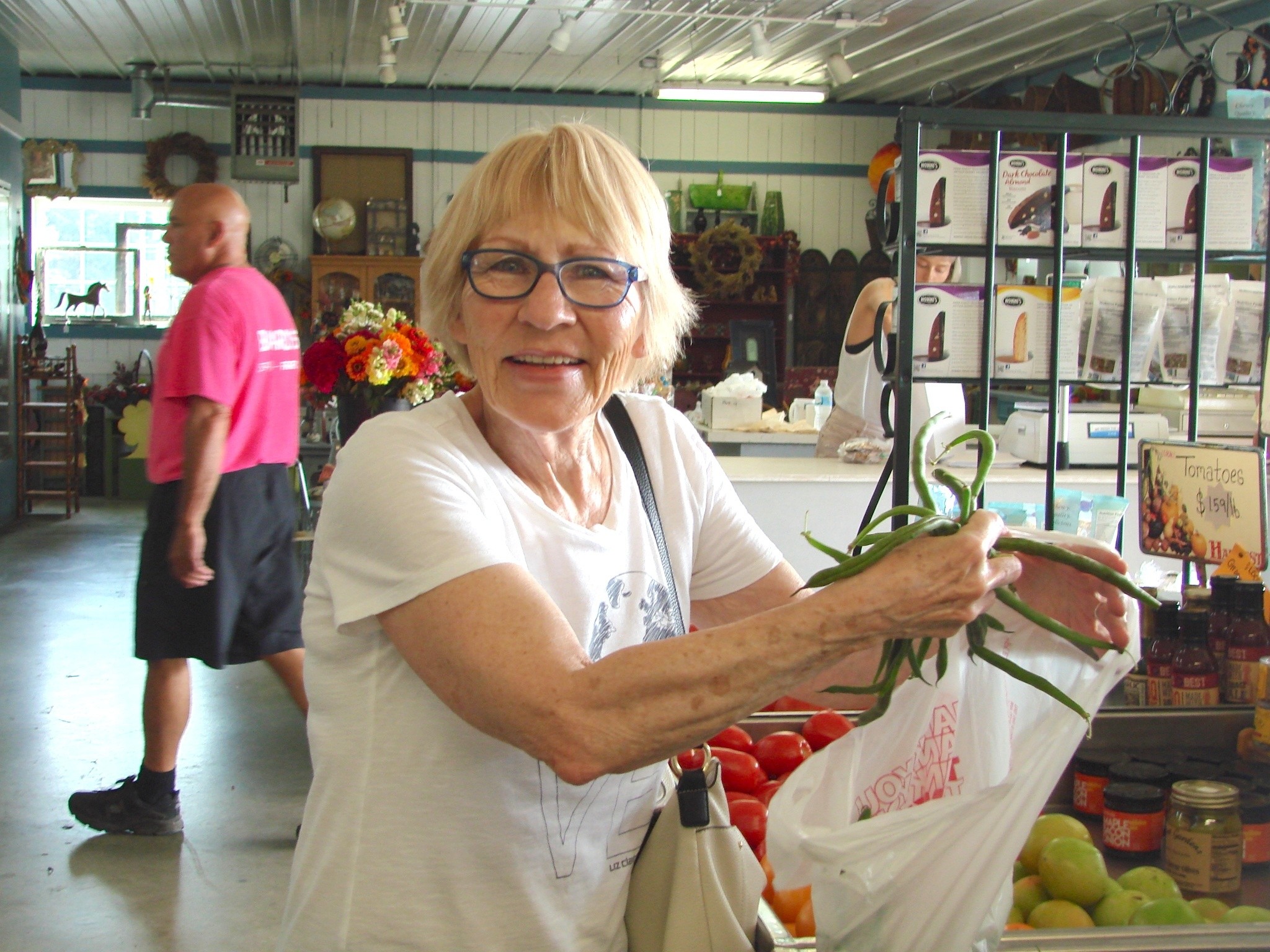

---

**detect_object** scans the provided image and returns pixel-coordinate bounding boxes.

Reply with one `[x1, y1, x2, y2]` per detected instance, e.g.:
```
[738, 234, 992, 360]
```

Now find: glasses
[459, 248, 643, 309]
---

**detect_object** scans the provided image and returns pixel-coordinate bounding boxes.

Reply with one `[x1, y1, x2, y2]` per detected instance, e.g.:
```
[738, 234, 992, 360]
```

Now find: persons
[276, 124, 1128, 952]
[67, 183, 307, 843]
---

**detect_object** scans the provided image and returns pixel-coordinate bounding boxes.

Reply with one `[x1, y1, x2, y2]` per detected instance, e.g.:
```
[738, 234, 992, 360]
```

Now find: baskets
[92, 349, 157, 416]
[689, 170, 753, 209]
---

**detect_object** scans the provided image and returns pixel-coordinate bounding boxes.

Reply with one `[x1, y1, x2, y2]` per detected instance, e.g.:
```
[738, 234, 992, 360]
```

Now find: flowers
[85, 362, 151, 404]
[301, 296, 442, 409]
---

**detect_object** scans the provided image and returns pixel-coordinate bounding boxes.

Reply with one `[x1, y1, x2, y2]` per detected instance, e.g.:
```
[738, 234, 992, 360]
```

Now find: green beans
[794, 412, 1162, 740]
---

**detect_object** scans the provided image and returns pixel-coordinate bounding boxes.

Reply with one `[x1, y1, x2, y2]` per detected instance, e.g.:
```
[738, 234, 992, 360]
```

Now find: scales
[997, 401, 1169, 463]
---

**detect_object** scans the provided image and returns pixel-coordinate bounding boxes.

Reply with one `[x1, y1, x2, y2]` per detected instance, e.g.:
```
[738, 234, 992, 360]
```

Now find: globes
[312, 197, 356, 255]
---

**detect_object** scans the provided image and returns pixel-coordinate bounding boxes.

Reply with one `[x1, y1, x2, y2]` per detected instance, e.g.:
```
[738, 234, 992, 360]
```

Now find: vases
[337, 391, 411, 446]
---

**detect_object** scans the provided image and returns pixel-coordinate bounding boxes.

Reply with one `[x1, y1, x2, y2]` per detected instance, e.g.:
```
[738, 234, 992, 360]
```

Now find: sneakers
[68, 776, 184, 837]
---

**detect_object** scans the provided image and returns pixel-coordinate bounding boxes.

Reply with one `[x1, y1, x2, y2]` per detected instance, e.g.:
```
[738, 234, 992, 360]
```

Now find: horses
[55, 281, 110, 324]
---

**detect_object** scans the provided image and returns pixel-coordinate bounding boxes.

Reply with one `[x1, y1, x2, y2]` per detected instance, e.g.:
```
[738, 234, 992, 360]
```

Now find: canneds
[1072, 656, 1269, 904]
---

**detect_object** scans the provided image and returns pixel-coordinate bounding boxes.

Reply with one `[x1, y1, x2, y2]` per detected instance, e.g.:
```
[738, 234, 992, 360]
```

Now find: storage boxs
[702, 389, 763, 429]
[918, 149, 1253, 379]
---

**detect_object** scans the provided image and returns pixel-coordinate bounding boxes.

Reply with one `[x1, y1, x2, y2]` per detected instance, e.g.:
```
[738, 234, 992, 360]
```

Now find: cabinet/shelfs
[891, 104, 1270, 584]
[670, 232, 794, 385]
[16, 333, 78, 519]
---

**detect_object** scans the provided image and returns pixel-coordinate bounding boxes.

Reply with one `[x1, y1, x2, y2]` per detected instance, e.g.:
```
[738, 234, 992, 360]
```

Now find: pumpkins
[1149, 500, 1207, 558]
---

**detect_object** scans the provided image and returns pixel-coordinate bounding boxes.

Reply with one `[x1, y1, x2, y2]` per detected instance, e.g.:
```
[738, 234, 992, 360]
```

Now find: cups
[789, 398, 815, 426]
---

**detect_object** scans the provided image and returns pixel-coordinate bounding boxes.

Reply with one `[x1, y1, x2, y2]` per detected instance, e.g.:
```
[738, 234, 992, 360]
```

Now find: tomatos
[674, 708, 856, 938]
[1004, 811, 1270, 928]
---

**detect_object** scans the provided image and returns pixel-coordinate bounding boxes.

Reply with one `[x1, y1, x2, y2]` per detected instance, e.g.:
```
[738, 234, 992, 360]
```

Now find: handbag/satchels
[909, 382, 966, 465]
[625, 756, 766, 952]
[766, 522, 1141, 952]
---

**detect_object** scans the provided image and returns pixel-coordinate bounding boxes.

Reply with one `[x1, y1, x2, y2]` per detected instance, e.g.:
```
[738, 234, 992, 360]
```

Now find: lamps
[749, 22, 772, 59]
[653, 84, 829, 103]
[828, 53, 853, 84]
[548, 16, 576, 52]
[377, 5, 409, 83]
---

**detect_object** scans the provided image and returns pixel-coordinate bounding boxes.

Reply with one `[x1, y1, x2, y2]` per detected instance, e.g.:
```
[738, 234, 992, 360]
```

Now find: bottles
[761, 191, 785, 237]
[1120, 574, 1270, 708]
[814, 379, 832, 431]
[694, 208, 707, 234]
